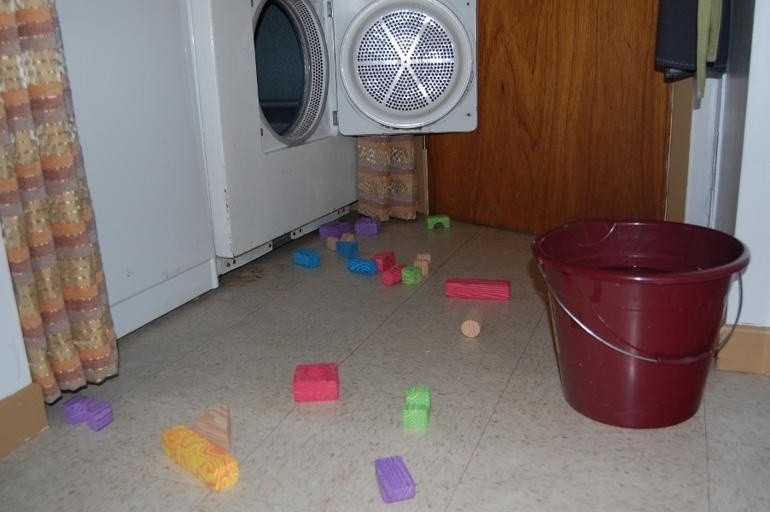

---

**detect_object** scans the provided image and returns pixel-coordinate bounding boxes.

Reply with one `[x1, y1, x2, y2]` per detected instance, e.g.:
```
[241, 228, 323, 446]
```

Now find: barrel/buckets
[531, 218, 750, 431]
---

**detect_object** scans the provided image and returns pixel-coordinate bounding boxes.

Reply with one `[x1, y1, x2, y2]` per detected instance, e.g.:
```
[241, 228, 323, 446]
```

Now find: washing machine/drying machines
[187, 1, 478, 276]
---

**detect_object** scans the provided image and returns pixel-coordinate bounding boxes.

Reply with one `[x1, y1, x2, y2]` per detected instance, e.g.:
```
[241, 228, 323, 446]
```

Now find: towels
[653, 0, 729, 83]
